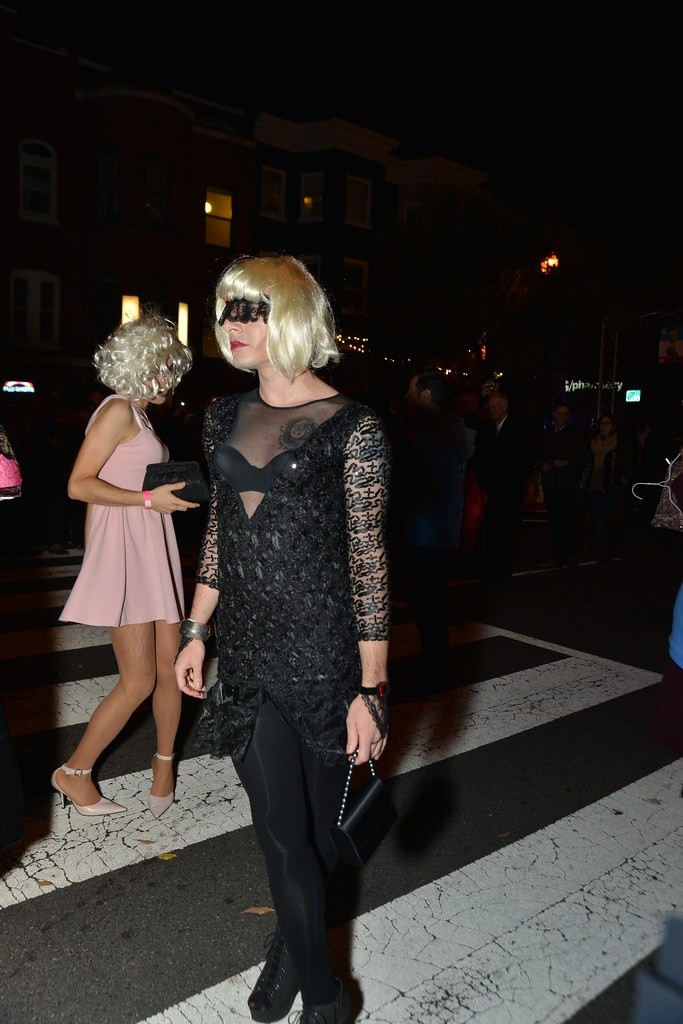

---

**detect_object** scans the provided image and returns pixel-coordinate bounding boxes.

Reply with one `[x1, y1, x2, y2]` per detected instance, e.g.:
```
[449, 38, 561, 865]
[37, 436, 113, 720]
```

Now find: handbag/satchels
[650, 452, 683, 532]
[332, 753, 398, 867]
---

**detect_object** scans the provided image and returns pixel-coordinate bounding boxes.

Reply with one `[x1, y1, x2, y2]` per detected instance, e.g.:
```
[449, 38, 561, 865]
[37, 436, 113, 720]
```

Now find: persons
[0, 386, 219, 558]
[385, 366, 667, 698]
[173, 254, 392, 1024]
[50, 316, 202, 820]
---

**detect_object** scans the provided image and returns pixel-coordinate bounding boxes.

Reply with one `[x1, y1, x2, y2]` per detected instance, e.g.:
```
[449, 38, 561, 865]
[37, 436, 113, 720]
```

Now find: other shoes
[399, 666, 418, 680]
[248, 932, 298, 1023]
[300, 977, 352, 1024]
[401, 685, 440, 697]
[537, 556, 557, 568]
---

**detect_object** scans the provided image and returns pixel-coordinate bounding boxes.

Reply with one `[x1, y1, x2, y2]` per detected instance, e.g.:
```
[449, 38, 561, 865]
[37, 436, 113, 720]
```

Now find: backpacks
[462, 458, 488, 540]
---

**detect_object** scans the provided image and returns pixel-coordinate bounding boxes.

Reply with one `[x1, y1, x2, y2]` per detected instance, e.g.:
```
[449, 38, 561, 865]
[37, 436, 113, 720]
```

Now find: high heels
[51, 763, 128, 816]
[147, 752, 175, 819]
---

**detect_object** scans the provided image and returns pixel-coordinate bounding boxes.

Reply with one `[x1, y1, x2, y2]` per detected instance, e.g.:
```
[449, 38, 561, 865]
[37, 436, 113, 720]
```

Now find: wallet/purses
[141, 461, 210, 504]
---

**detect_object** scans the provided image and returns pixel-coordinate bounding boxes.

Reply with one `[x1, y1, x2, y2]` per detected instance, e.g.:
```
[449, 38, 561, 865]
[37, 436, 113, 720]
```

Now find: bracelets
[143, 490, 152, 510]
[358, 681, 391, 697]
[177, 618, 211, 641]
